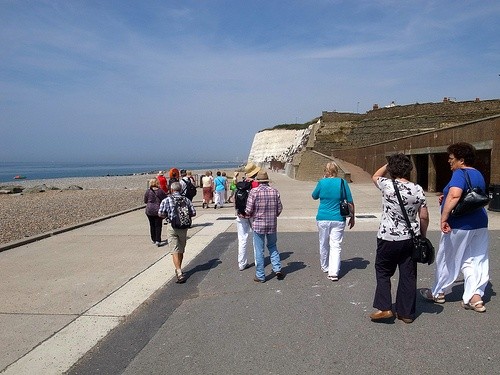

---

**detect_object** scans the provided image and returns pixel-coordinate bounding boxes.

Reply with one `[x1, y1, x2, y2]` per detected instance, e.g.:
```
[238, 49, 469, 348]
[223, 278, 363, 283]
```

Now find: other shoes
[253, 271, 338, 282]
[369, 310, 413, 323]
[174, 267, 188, 283]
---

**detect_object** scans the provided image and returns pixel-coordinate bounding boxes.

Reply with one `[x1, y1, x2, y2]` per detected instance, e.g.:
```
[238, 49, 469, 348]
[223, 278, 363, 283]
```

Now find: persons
[311, 161, 355, 281]
[144, 178, 168, 247]
[369, 154, 429, 324]
[167, 172, 179, 191]
[421, 141, 491, 312]
[244, 169, 285, 283]
[202, 170, 239, 209]
[157, 171, 168, 193]
[235, 163, 261, 271]
[180, 169, 196, 200]
[157, 182, 196, 283]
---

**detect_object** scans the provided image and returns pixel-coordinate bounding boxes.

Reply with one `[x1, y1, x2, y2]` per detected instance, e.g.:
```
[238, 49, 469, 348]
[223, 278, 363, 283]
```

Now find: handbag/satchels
[340, 178, 354, 217]
[447, 168, 489, 218]
[413, 235, 435, 266]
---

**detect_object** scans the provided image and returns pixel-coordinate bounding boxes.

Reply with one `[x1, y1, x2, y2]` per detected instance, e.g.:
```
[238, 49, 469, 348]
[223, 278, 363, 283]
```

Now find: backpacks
[181, 177, 197, 201]
[169, 195, 192, 229]
[235, 179, 253, 216]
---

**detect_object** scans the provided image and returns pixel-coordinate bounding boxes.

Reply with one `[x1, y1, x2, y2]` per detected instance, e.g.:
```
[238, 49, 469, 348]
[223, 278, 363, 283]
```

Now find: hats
[254, 169, 271, 183]
[244, 163, 261, 177]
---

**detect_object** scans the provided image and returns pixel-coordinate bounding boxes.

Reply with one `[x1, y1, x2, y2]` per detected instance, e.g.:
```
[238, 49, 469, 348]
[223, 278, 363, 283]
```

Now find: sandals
[420, 287, 445, 303]
[460, 300, 486, 312]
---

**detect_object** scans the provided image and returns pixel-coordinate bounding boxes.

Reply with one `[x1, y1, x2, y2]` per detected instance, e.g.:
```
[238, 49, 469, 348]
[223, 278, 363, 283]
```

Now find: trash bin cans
[487, 184, 500, 212]
